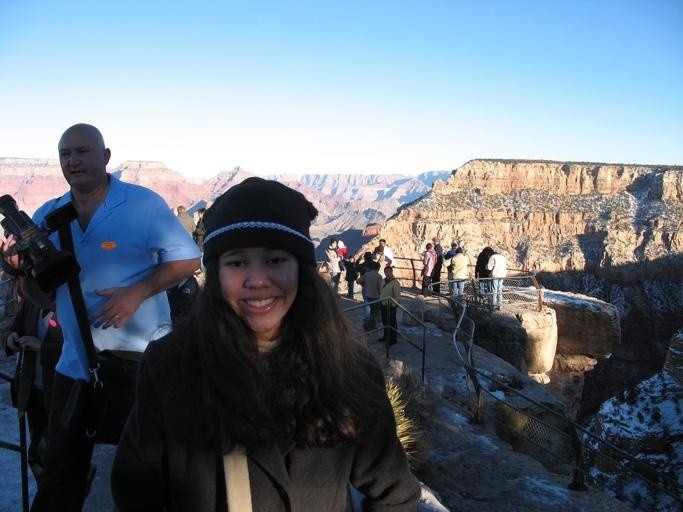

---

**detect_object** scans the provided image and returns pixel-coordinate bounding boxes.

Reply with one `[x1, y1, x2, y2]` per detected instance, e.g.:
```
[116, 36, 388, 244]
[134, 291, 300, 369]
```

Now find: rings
[115, 314, 121, 321]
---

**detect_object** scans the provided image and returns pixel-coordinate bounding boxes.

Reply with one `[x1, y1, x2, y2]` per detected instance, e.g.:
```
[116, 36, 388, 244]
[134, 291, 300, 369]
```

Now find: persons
[432, 244, 444, 293]
[444, 243, 457, 295]
[1, 280, 98, 501]
[444, 247, 469, 294]
[422, 243, 437, 296]
[475, 247, 494, 304]
[0, 122, 202, 510]
[110, 176, 422, 510]
[175, 206, 196, 240]
[324, 237, 401, 347]
[484, 251, 507, 311]
[191, 208, 206, 274]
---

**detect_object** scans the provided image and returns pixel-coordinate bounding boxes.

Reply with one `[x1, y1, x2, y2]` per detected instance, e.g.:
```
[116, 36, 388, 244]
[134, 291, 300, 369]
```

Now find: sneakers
[83, 464, 97, 497]
[378, 336, 396, 345]
[194, 268, 204, 277]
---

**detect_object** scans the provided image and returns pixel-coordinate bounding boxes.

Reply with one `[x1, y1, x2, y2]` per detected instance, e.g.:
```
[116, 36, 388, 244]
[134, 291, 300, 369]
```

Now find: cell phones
[13, 338, 20, 348]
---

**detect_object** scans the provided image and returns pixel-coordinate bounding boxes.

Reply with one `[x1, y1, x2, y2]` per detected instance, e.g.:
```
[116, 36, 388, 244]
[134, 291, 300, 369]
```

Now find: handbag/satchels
[10, 378, 25, 408]
[62, 348, 141, 444]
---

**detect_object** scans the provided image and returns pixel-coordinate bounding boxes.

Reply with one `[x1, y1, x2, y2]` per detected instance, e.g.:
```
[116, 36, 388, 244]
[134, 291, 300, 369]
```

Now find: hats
[377, 245, 385, 251]
[338, 240, 344, 247]
[363, 251, 372, 257]
[204, 176, 318, 266]
